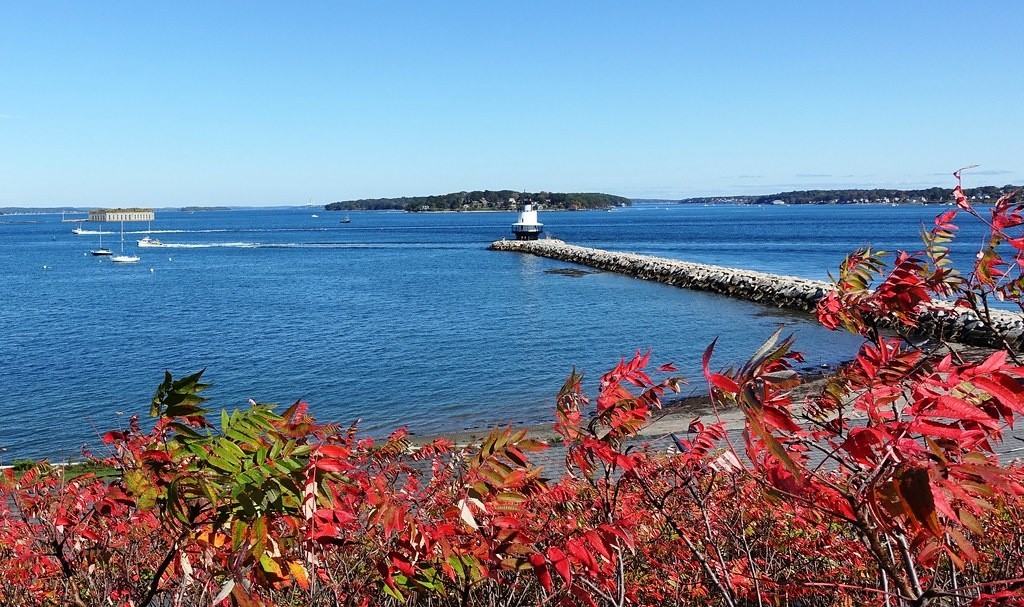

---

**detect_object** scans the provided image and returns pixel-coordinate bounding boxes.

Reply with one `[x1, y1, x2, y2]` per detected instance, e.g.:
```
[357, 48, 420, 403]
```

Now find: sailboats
[136, 215, 165, 248]
[71, 219, 82, 235]
[110, 219, 140, 262]
[90, 225, 114, 256]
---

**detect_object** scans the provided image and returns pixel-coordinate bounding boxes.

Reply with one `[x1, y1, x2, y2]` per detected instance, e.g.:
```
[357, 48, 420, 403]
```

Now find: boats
[340, 217, 351, 223]
